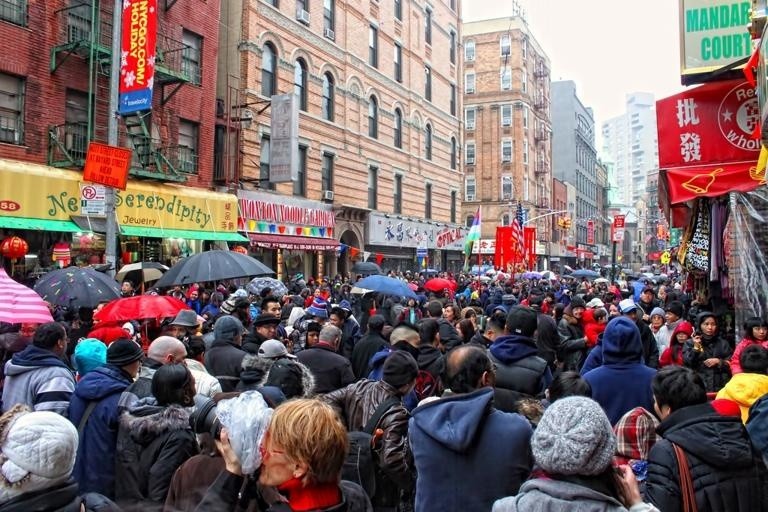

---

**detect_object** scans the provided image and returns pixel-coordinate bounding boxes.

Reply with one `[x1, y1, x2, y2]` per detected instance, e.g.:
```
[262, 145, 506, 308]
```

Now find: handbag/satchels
[341, 398, 400, 499]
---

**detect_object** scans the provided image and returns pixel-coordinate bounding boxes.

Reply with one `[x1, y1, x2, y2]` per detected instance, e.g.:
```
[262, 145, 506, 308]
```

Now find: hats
[590, 298, 606, 308]
[570, 295, 586, 310]
[0, 404, 78, 503]
[304, 298, 327, 317]
[531, 396, 617, 477]
[107, 340, 143, 367]
[229, 289, 249, 298]
[383, 350, 418, 387]
[650, 301, 683, 320]
[74, 339, 106, 377]
[170, 309, 320, 359]
[506, 306, 536, 336]
[617, 298, 637, 313]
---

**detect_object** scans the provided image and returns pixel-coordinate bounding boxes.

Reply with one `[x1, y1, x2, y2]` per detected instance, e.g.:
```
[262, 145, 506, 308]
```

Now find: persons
[0, 237, 768, 512]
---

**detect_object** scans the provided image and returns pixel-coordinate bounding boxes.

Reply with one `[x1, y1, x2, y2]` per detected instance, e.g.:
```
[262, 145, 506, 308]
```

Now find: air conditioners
[323, 191, 334, 201]
[325, 27, 335, 41]
[296, 9, 311, 24]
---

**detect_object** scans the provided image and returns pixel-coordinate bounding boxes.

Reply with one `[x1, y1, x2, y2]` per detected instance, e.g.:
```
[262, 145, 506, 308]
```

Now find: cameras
[192, 400, 237, 443]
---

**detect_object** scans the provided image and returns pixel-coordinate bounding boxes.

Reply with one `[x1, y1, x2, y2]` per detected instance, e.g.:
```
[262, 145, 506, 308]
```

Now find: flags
[510, 201, 528, 270]
[464, 204, 481, 275]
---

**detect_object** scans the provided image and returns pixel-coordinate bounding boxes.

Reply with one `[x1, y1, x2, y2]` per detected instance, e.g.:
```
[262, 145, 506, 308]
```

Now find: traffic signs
[613, 215, 624, 229]
[79, 180, 106, 201]
[80, 198, 106, 218]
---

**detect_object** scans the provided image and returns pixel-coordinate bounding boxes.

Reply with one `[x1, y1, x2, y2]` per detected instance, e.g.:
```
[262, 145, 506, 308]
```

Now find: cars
[592, 263, 600, 266]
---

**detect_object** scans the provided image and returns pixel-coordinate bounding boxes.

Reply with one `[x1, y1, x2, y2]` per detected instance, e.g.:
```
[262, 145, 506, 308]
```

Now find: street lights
[523, 209, 569, 225]
